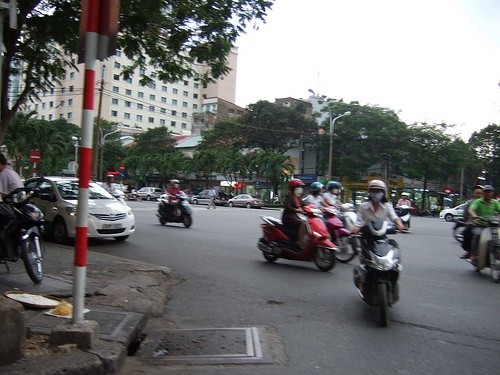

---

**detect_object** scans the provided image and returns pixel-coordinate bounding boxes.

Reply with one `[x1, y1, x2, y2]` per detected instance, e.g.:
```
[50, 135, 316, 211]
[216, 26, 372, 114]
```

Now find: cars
[159, 191, 193, 202]
[190, 189, 227, 205]
[23, 176, 135, 242]
[228, 194, 265, 209]
[137, 187, 164, 200]
[440, 202, 468, 222]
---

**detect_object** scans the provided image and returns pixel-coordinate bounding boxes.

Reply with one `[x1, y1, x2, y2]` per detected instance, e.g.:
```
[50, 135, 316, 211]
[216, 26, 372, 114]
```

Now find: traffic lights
[444, 189, 451, 194]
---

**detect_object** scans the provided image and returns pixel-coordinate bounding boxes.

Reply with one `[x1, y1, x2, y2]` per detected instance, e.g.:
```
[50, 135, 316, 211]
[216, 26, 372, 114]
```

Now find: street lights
[308, 89, 351, 181]
[93, 121, 123, 183]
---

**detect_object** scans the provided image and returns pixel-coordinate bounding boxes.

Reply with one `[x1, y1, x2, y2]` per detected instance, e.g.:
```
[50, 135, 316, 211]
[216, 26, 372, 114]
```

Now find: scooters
[156, 194, 193, 228]
[395, 206, 416, 230]
[257, 203, 358, 273]
[346, 219, 407, 327]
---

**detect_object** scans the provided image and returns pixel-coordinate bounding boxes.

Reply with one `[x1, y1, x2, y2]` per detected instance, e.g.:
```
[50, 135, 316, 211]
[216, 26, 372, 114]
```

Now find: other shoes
[461, 251, 471, 259]
[471, 255, 477, 262]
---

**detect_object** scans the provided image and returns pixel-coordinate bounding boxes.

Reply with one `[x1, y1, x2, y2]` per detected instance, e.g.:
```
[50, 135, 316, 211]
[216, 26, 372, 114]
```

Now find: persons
[351, 180, 405, 264]
[459, 185, 500, 263]
[273, 195, 279, 204]
[410, 194, 417, 205]
[302, 182, 328, 209]
[207, 186, 216, 209]
[164, 179, 186, 203]
[0, 152, 26, 202]
[322, 181, 344, 206]
[127, 183, 136, 193]
[219, 189, 227, 200]
[437, 195, 444, 210]
[282, 179, 305, 253]
[430, 202, 437, 218]
[184, 187, 190, 194]
[102, 175, 117, 195]
[397, 192, 414, 228]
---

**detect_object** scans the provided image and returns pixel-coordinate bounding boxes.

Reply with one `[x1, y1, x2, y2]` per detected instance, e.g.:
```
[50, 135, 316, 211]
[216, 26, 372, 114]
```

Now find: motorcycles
[0, 176, 44, 283]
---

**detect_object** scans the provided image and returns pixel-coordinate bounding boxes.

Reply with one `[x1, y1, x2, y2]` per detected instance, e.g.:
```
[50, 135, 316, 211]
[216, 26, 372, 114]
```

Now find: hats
[483, 185, 494, 191]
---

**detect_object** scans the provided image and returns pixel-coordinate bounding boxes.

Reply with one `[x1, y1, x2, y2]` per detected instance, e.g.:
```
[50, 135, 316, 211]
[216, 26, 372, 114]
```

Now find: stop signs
[30, 152, 40, 163]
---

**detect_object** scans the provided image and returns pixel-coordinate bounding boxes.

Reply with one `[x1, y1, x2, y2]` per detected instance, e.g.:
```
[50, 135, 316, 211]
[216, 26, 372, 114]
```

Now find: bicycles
[453, 218, 465, 242]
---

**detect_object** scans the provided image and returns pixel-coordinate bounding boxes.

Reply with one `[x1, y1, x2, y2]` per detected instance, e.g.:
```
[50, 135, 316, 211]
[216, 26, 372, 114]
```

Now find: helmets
[311, 182, 324, 191]
[288, 179, 306, 187]
[107, 174, 114, 178]
[368, 180, 387, 193]
[328, 181, 339, 188]
[401, 192, 407, 196]
[170, 179, 180, 183]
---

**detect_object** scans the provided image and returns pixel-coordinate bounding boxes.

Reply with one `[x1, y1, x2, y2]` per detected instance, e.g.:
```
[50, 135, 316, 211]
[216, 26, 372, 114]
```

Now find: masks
[370, 192, 384, 202]
[174, 184, 179, 188]
[294, 188, 303, 194]
[331, 189, 338, 194]
